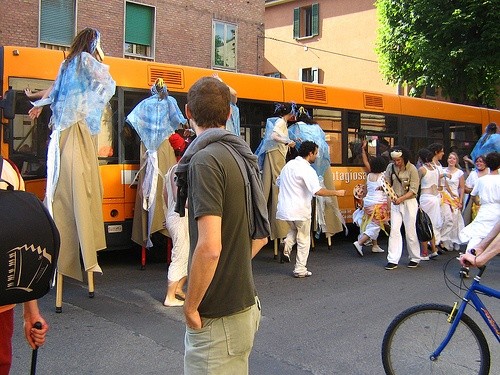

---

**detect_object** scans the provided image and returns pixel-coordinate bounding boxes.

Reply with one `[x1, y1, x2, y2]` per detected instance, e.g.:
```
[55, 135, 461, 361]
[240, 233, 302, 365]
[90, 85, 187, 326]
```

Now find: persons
[275, 141, 346, 278]
[1, 155, 48, 375]
[352, 121, 500, 271]
[257, 102, 297, 223]
[162, 135, 194, 307]
[24, 25, 116, 228]
[181, 77, 268, 375]
[126, 77, 189, 217]
[212, 74, 241, 135]
[286, 106, 337, 218]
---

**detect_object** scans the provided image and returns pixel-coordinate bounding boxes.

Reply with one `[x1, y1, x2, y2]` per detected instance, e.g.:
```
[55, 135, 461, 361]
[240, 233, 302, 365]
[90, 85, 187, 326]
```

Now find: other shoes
[455, 243, 460, 251]
[175, 291, 185, 299]
[459, 267, 469, 278]
[294, 271, 312, 277]
[280, 242, 290, 262]
[164, 299, 184, 307]
[437, 250, 442, 255]
[420, 256, 429, 260]
[447, 247, 453, 252]
[438, 243, 448, 252]
[384, 263, 398, 270]
[408, 261, 418, 268]
[428, 252, 439, 258]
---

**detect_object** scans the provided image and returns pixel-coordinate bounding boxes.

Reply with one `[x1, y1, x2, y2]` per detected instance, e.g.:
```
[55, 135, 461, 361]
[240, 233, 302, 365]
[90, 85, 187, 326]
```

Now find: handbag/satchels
[416, 207, 434, 242]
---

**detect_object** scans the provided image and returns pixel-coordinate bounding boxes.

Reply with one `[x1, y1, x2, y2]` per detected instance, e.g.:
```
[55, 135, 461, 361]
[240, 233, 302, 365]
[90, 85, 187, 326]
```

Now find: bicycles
[380, 249, 500, 375]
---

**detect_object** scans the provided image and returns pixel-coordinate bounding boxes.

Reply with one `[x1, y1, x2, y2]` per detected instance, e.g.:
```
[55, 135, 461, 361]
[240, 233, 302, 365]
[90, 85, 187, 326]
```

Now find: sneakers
[372, 245, 385, 253]
[353, 241, 363, 256]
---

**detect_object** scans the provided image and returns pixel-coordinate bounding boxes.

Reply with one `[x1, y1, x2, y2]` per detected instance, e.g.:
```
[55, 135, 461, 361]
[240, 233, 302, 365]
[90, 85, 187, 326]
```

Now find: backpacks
[0, 156, 61, 307]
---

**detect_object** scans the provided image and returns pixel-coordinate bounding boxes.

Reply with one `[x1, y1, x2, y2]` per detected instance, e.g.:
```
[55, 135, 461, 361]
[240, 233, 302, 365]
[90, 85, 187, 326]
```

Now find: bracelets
[472, 255, 479, 267]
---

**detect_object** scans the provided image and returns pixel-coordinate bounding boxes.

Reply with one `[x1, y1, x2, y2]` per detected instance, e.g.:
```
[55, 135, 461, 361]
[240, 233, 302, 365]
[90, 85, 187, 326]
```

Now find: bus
[0, 45, 500, 267]
